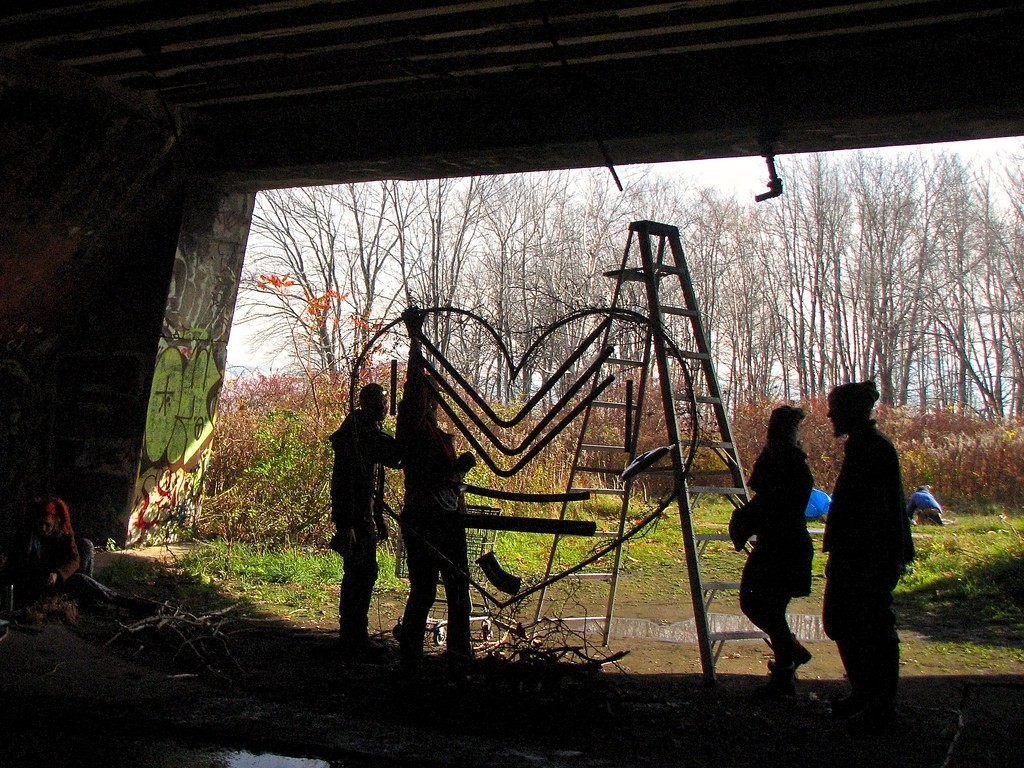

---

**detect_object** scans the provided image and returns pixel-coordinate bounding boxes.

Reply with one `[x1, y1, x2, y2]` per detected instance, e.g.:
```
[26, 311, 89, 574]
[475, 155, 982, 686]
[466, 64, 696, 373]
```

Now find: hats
[768, 406, 806, 442]
[830, 380, 880, 409]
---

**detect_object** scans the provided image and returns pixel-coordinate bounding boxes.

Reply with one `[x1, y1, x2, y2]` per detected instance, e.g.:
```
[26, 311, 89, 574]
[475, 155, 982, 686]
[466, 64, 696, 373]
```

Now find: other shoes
[751, 640, 812, 695]
[833, 687, 897, 726]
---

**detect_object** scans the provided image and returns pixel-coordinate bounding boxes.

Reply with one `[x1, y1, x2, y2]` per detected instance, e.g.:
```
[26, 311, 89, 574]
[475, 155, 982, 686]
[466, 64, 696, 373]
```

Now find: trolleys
[393, 505, 505, 646]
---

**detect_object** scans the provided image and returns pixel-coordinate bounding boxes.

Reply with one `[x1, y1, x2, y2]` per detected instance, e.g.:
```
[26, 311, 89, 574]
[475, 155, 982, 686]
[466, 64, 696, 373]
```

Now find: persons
[908, 485, 943, 525]
[329, 306, 476, 657]
[730, 406, 814, 701]
[26, 495, 111, 601]
[824, 381, 916, 733]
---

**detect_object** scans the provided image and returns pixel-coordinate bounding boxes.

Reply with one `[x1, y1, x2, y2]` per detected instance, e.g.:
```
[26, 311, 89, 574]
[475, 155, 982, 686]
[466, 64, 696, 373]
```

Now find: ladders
[529, 220, 800, 703]
